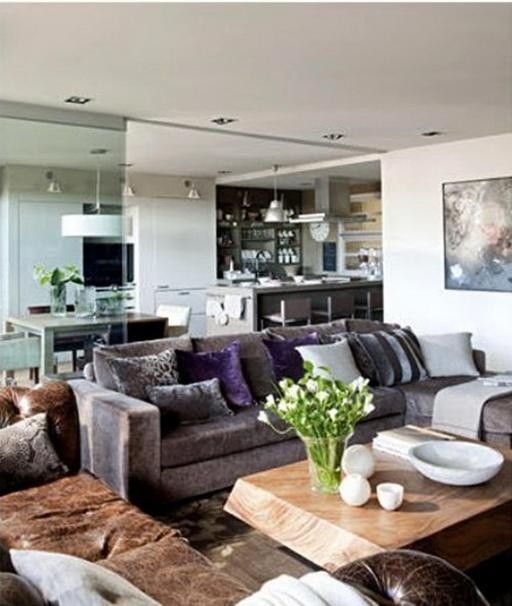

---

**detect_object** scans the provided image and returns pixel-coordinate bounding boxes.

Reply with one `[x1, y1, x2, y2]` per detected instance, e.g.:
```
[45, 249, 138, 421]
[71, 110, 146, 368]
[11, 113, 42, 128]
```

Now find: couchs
[66, 317, 512, 501]
[0, 373, 491, 606]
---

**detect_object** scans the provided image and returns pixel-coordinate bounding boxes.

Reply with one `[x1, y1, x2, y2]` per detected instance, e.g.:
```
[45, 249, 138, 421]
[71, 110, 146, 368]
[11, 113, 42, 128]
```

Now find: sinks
[255, 272, 270, 277]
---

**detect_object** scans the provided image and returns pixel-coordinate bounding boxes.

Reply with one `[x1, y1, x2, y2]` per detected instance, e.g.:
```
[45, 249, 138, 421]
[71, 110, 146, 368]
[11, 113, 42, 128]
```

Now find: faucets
[255, 251, 266, 268]
[256, 249, 273, 269]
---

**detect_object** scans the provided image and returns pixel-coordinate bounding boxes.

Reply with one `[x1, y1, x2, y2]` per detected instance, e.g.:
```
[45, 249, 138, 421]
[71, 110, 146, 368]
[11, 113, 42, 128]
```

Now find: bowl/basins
[409, 439, 507, 486]
[258, 277, 281, 284]
[291, 274, 306, 282]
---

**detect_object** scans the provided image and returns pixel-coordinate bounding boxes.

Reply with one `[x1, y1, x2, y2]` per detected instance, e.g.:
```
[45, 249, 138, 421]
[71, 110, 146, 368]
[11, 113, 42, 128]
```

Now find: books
[372, 423, 457, 461]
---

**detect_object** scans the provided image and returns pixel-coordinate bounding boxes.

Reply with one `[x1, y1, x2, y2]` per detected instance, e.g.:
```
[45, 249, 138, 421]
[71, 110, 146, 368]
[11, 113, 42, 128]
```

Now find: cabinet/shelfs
[217, 187, 302, 280]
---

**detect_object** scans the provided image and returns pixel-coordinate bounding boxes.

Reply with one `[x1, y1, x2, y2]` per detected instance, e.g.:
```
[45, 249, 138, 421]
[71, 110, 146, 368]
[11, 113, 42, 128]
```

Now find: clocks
[309, 219, 330, 242]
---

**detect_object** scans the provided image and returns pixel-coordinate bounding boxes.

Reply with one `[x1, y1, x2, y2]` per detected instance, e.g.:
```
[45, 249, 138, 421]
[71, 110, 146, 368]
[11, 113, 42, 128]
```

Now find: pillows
[0, 411, 69, 493]
[8, 548, 164, 606]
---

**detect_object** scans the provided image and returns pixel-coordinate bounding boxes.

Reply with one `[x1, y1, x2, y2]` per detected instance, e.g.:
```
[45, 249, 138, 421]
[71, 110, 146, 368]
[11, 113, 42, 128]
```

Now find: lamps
[122, 184, 135, 197]
[186, 183, 201, 199]
[46, 175, 64, 193]
[263, 164, 290, 222]
[61, 147, 134, 237]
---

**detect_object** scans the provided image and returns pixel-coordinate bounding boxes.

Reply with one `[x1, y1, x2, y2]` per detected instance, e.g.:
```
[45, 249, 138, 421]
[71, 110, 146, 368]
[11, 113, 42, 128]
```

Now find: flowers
[258, 362, 376, 490]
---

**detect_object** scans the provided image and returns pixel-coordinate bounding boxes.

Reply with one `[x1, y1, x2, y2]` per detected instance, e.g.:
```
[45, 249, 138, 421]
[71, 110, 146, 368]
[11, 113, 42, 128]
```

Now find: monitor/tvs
[83, 243, 133, 289]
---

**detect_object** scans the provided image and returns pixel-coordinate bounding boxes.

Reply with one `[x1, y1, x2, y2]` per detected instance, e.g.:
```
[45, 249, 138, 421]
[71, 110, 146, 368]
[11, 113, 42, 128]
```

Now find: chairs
[261, 288, 383, 328]
[0, 304, 193, 385]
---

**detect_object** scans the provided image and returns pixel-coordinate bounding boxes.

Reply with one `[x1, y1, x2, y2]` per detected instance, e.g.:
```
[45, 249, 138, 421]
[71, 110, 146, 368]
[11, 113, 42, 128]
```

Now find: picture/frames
[442, 176, 512, 292]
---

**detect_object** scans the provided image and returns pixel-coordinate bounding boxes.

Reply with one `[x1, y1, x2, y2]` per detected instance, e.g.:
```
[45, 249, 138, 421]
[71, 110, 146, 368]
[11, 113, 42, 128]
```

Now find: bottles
[219, 206, 300, 265]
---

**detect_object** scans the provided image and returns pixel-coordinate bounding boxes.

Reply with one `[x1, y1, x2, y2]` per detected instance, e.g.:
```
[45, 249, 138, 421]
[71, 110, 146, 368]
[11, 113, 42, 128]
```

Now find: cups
[378, 480, 406, 511]
[74, 285, 97, 316]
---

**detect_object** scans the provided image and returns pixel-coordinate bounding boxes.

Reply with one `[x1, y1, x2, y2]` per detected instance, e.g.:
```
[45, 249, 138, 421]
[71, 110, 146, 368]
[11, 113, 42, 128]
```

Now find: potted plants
[32, 263, 85, 316]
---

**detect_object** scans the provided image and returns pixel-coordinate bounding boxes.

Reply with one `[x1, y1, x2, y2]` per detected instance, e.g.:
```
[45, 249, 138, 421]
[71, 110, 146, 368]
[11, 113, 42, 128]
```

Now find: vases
[296, 432, 354, 493]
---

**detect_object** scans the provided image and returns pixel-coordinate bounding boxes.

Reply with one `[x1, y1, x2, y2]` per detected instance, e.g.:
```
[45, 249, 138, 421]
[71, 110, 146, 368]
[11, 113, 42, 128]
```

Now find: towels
[206, 293, 244, 326]
[235, 570, 368, 606]
